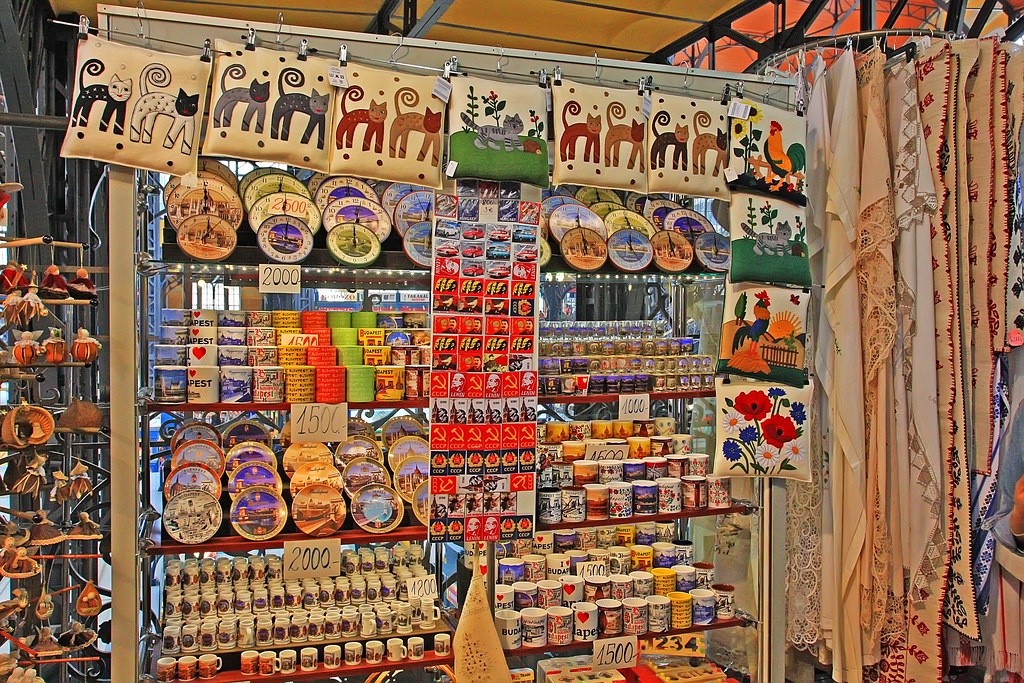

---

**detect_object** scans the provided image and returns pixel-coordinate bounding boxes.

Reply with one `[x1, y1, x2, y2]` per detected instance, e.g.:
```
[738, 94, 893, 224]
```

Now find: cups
[154, 309, 430, 406]
[157, 544, 449, 682]
[463, 416, 736, 650]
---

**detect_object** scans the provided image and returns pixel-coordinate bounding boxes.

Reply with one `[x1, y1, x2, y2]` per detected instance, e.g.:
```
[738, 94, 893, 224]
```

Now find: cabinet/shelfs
[138, 169, 771, 683]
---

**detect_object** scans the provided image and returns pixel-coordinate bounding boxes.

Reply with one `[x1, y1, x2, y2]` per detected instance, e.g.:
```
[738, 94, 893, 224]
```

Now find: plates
[162, 417, 429, 540]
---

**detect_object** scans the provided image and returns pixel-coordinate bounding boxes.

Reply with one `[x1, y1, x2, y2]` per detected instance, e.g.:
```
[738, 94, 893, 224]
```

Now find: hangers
[801, 28, 931, 77]
[242, 12, 314, 61]
[726, 71, 807, 117]
[531, 50, 660, 97]
[47, 0, 232, 63]
[313, 32, 468, 82]
[624, 61, 743, 105]
[450, 44, 547, 88]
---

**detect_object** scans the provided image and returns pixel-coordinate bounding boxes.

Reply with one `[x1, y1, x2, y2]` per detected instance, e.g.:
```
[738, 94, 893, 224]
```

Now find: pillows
[550, 78, 648, 194]
[729, 188, 812, 287]
[448, 76, 550, 190]
[714, 377, 815, 483]
[716, 272, 811, 388]
[59, 33, 212, 178]
[648, 90, 732, 202]
[728, 98, 807, 206]
[329, 64, 446, 190]
[202, 37, 341, 175]
